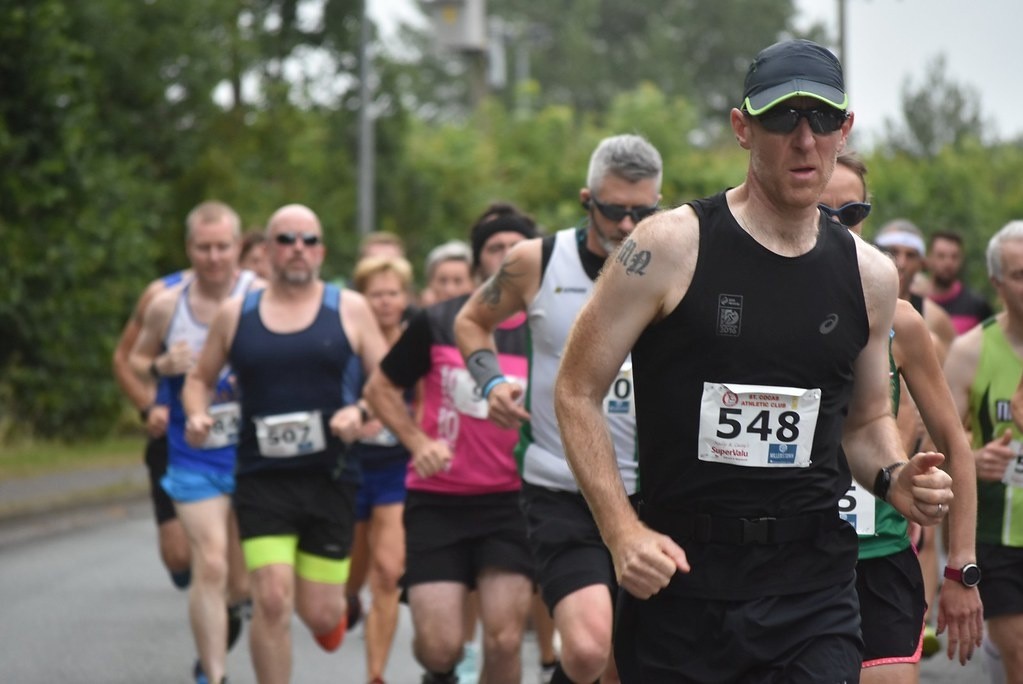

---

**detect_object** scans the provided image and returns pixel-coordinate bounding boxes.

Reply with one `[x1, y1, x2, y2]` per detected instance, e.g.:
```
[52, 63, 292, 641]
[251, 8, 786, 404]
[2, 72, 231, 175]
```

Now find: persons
[115, 36, 1022, 684]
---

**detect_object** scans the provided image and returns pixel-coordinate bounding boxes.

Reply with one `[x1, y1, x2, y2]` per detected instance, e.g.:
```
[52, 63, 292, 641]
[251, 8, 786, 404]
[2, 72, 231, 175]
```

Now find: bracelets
[483, 377, 505, 400]
[149, 359, 161, 379]
[138, 402, 155, 422]
[354, 401, 369, 426]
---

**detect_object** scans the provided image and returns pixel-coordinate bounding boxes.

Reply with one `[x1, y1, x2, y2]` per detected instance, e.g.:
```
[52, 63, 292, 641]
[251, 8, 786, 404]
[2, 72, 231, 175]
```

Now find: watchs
[873, 461, 910, 502]
[943, 562, 983, 588]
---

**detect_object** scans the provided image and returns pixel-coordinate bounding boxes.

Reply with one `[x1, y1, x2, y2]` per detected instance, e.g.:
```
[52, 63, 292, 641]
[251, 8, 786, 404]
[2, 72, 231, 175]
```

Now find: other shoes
[226, 602, 242, 650]
[455, 642, 479, 683]
[922, 621, 940, 657]
[315, 612, 348, 655]
[540, 648, 559, 684]
[424, 669, 458, 684]
[980, 634, 1006, 684]
[347, 596, 361, 629]
[172, 570, 190, 588]
[194, 658, 227, 684]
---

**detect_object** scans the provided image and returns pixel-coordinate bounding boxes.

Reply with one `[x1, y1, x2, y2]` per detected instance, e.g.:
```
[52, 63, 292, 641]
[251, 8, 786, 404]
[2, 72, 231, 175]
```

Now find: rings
[937, 502, 943, 513]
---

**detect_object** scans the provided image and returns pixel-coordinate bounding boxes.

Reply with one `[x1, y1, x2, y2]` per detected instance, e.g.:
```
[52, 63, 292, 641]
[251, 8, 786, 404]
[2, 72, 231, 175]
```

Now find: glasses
[592, 195, 658, 224]
[743, 105, 850, 134]
[277, 232, 317, 246]
[819, 201, 872, 228]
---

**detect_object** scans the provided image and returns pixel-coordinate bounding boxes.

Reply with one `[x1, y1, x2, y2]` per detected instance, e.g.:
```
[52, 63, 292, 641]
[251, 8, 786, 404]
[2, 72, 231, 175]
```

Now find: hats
[741, 38, 848, 116]
